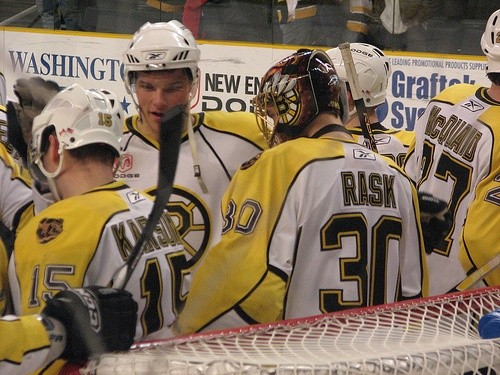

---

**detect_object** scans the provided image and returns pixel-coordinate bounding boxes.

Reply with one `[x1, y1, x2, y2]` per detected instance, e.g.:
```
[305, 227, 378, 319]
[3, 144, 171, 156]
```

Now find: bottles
[479, 307, 500, 336]
[482, 317, 500, 339]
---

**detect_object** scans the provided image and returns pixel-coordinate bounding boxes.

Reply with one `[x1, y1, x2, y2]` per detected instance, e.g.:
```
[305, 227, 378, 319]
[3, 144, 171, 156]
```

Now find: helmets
[0, 71, 8, 107]
[122, 18, 201, 102]
[31, 83, 124, 163]
[256, 49, 341, 144]
[480, 9, 500, 75]
[325, 42, 392, 107]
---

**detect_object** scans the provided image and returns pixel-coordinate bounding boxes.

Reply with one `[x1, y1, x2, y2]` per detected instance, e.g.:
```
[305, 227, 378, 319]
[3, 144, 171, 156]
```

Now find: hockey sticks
[338, 42, 380, 152]
[113, 103, 186, 291]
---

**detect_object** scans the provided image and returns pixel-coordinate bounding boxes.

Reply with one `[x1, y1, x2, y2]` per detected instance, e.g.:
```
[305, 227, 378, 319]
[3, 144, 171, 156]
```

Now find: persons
[36, 0, 421, 46]
[6, 82, 191, 350]
[0, 76, 64, 317]
[110, 18, 273, 334]
[401, 8, 500, 297]
[0, 285, 139, 375]
[326, 43, 409, 170]
[144, 48, 428, 346]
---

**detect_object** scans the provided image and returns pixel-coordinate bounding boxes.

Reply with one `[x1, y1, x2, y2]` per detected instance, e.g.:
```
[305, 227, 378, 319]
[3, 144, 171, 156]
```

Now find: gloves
[42, 284, 138, 362]
